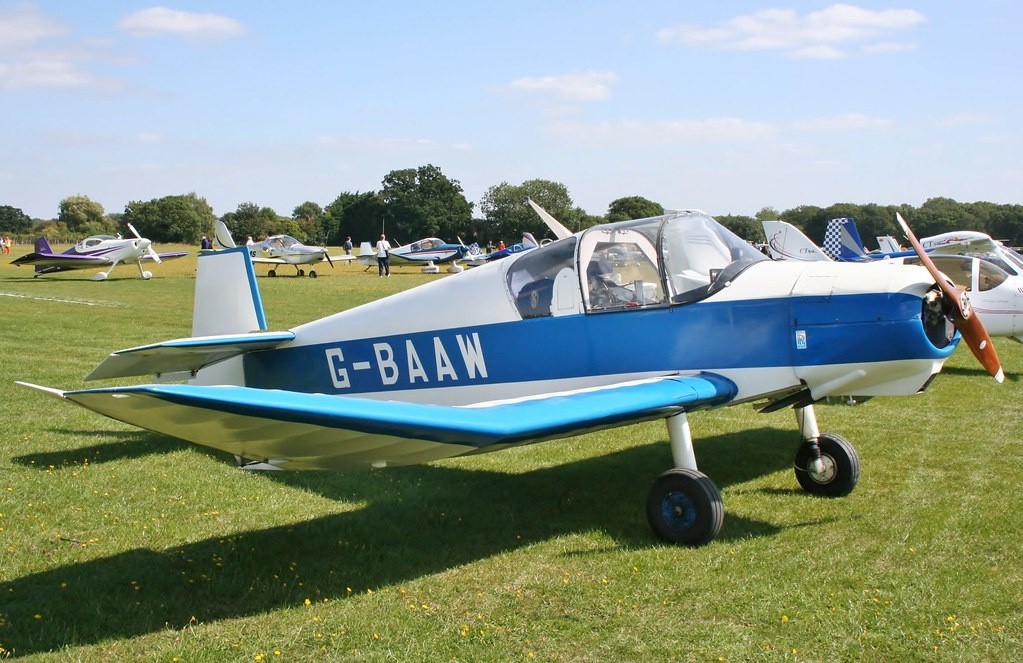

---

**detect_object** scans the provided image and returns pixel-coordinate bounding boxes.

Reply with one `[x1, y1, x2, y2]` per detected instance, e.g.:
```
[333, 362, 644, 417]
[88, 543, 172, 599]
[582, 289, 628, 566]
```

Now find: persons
[413, 243, 420, 250]
[247, 237, 254, 246]
[343, 236, 353, 265]
[486, 241, 496, 253]
[752, 241, 772, 258]
[200, 235, 213, 249]
[498, 240, 505, 251]
[376, 234, 391, 277]
[0, 236, 12, 255]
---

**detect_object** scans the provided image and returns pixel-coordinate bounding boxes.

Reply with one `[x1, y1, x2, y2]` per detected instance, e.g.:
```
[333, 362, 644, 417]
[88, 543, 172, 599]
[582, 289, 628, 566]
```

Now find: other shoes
[380, 275, 382, 277]
[387, 273, 390, 278]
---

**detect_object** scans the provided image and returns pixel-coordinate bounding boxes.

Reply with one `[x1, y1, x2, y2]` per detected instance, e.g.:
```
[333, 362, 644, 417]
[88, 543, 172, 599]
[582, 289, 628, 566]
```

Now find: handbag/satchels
[385, 250, 389, 258]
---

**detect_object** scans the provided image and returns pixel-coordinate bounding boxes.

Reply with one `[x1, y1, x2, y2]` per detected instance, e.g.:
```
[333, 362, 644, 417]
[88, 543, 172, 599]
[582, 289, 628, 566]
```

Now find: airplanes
[19, 195, 1005, 550]
[760, 211, 1023, 337]
[357, 236, 469, 273]
[9, 222, 188, 282]
[197, 220, 358, 278]
